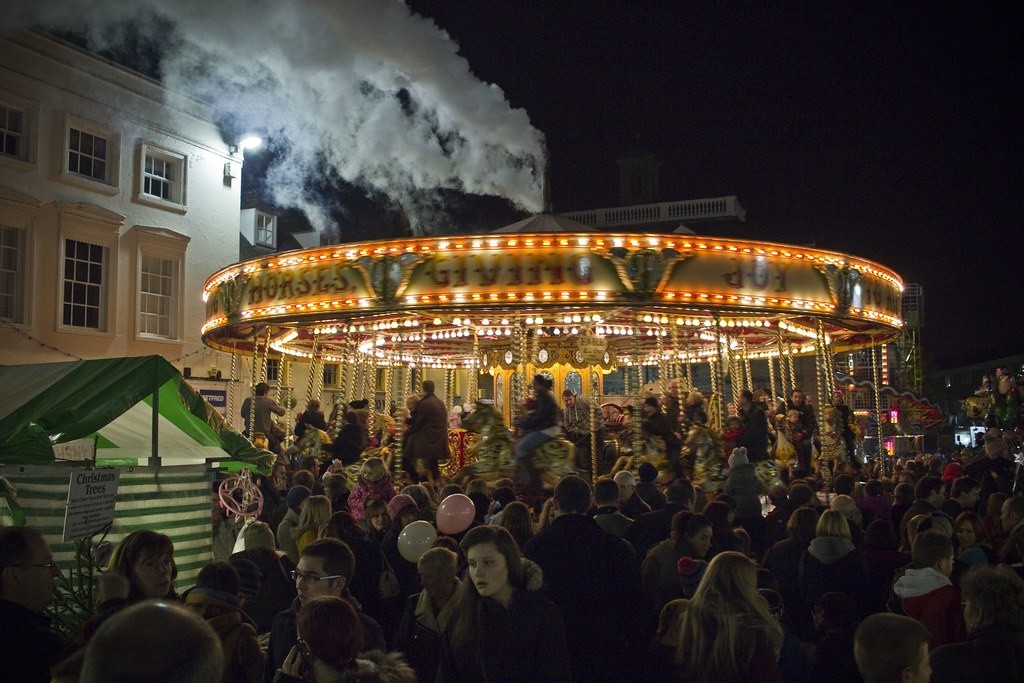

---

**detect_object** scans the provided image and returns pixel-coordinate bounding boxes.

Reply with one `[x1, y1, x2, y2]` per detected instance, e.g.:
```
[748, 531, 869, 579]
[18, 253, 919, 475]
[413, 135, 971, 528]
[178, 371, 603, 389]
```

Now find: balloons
[436, 494, 476, 535]
[398, 521, 436, 562]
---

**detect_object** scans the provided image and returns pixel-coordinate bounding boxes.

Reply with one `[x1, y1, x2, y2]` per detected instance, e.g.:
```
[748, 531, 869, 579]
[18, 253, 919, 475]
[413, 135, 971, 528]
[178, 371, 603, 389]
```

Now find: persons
[2, 375, 1023, 682]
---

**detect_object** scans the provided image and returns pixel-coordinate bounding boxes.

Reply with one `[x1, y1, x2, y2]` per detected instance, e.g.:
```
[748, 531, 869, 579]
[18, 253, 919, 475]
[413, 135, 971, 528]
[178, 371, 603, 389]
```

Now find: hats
[387, 494, 418, 522]
[702, 500, 730, 527]
[941, 464, 965, 480]
[286, 485, 311, 508]
[756, 587, 784, 614]
[677, 558, 711, 596]
[232, 558, 262, 596]
[726, 446, 749, 468]
[982, 434, 1010, 455]
[638, 463, 658, 483]
[323, 473, 348, 494]
[789, 484, 813, 504]
[864, 518, 901, 550]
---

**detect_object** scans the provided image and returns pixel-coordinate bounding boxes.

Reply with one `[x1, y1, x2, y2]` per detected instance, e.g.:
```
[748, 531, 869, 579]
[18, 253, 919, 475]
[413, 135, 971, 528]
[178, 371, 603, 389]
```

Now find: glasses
[961, 600, 974, 609]
[289, 569, 340, 585]
[11, 560, 56, 570]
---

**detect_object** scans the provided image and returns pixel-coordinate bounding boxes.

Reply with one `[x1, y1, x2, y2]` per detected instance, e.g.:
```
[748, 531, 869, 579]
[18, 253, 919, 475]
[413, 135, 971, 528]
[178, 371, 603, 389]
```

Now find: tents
[0, 353, 277, 617]
